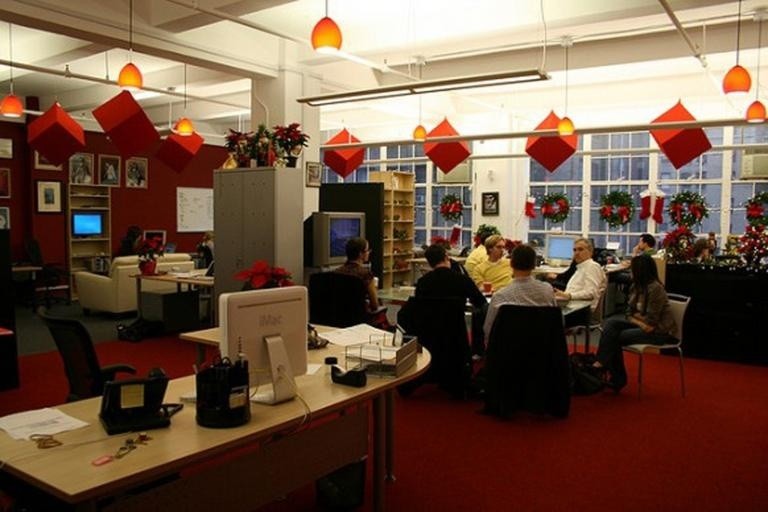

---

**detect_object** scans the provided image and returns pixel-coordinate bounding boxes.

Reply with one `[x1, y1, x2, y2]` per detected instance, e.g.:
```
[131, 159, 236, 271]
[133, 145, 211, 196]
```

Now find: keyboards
[180, 391, 197, 403]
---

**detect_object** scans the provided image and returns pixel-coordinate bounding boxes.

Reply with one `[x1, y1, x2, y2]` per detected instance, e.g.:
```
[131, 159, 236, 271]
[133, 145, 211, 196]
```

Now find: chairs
[309, 238, 694, 416]
[36, 307, 136, 406]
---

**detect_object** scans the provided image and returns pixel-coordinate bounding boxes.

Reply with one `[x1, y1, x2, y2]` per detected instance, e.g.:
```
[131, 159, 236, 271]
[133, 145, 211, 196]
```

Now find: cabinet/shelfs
[66, 183, 115, 305]
[369, 170, 417, 290]
[213, 167, 307, 328]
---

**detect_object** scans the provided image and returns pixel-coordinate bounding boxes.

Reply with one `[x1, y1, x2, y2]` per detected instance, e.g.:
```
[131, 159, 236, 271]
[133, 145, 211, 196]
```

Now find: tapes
[333, 364, 353, 377]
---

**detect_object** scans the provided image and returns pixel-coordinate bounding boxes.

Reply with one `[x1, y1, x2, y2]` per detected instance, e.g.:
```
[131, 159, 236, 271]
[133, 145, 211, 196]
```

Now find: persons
[104, 161, 117, 183]
[120, 226, 142, 256]
[335, 237, 379, 311]
[72, 158, 91, 183]
[484, 195, 496, 209]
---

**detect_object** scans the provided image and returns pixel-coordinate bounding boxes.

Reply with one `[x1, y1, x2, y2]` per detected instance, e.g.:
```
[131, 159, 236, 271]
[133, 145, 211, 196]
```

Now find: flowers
[660, 191, 768, 272]
[598, 189, 636, 229]
[438, 193, 464, 222]
[224, 122, 311, 168]
[541, 192, 570, 223]
[474, 223, 503, 247]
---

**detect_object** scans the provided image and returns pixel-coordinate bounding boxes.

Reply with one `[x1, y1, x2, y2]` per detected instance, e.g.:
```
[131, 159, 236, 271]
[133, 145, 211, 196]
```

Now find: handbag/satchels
[570, 350, 603, 396]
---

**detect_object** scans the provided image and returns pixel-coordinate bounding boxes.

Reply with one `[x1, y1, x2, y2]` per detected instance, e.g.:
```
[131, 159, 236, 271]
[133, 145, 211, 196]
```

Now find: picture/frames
[305, 161, 324, 187]
[33, 149, 150, 215]
[143, 229, 167, 247]
[482, 192, 500, 217]
[0, 168, 13, 200]
[0, 207, 11, 230]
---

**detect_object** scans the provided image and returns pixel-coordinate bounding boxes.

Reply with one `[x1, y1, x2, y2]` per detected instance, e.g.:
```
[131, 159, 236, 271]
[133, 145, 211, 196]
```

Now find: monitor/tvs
[303, 211, 366, 268]
[71, 213, 103, 238]
[218, 286, 308, 405]
[544, 234, 580, 267]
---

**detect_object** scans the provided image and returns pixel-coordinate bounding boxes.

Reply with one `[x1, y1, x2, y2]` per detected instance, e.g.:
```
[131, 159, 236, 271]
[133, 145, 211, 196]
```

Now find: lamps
[294, 0, 552, 107]
[411, 93, 428, 143]
[310, 0, 343, 55]
[557, 46, 576, 137]
[117, 0, 144, 93]
[175, 62, 195, 137]
[721, 1, 752, 97]
[745, 17, 767, 125]
[0, 20, 26, 119]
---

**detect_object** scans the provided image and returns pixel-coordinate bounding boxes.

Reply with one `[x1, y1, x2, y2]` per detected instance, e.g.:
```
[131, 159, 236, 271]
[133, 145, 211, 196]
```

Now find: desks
[0, 322, 432, 512]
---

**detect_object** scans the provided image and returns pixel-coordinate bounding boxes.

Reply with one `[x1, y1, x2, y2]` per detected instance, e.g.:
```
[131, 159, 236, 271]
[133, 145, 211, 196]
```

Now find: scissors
[209, 355, 231, 368]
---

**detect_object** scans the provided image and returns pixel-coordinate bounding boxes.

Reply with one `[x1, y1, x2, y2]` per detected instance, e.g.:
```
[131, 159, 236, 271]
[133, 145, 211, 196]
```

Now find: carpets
[0, 329, 767, 510]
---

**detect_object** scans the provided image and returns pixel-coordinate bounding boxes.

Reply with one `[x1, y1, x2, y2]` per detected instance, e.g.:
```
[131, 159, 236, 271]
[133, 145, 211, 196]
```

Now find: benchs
[2, 239, 217, 343]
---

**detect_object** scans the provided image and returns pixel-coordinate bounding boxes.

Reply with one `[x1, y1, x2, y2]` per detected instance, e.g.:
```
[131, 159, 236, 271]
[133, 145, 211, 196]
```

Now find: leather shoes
[600, 379, 626, 395]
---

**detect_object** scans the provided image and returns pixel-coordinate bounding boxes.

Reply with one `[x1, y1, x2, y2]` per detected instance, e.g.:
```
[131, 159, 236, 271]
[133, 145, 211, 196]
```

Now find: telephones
[99, 368, 168, 435]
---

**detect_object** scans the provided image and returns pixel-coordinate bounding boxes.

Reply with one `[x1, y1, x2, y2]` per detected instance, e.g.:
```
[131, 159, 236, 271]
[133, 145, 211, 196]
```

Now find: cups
[483, 281, 493, 294]
[195, 368, 251, 428]
[373, 277, 379, 287]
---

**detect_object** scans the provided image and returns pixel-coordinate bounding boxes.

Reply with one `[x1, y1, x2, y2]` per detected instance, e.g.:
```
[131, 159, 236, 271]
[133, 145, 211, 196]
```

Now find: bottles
[323, 355, 338, 382]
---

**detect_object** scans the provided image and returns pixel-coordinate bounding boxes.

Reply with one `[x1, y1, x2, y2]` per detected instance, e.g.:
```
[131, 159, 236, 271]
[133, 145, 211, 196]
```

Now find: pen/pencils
[192, 364, 207, 384]
[210, 360, 248, 384]
